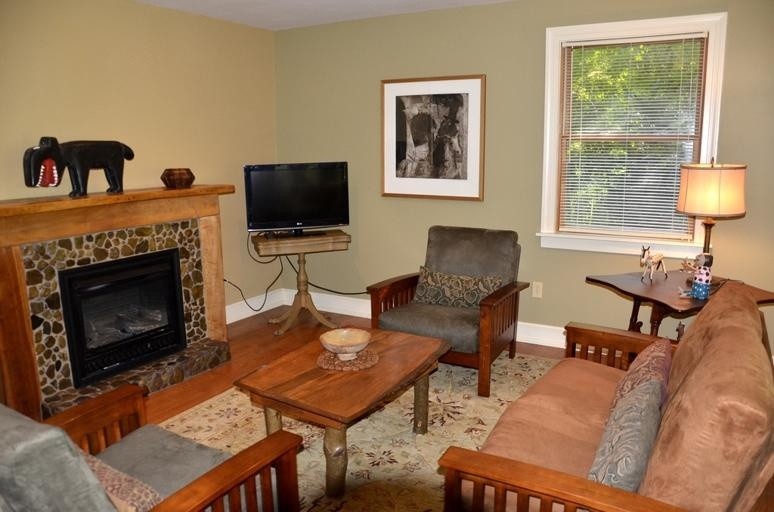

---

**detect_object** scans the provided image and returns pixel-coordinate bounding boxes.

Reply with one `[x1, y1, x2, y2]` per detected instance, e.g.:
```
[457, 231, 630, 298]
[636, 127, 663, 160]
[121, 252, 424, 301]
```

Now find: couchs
[436, 282, 774, 512]
[1, 383, 304, 511]
[362, 225, 531, 397]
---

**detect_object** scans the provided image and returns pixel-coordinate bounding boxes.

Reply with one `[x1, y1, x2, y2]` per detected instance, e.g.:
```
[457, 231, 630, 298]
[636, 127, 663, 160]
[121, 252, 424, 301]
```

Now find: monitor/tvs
[243, 160, 350, 238]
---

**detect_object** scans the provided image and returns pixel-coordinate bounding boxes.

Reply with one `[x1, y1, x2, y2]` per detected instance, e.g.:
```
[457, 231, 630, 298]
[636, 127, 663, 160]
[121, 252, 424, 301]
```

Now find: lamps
[673, 157, 748, 275]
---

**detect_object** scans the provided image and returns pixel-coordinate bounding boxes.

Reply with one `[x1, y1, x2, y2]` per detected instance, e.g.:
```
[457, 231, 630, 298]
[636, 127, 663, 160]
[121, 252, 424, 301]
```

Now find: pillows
[587, 379, 664, 492]
[608, 336, 671, 405]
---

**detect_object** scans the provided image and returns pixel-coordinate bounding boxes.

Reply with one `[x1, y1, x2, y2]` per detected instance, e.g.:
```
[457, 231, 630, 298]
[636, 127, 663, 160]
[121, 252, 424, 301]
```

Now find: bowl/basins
[318, 327, 372, 361]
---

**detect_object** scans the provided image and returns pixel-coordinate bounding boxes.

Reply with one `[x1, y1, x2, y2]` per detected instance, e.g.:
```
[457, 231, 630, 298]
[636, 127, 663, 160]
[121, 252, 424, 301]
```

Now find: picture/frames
[379, 73, 486, 204]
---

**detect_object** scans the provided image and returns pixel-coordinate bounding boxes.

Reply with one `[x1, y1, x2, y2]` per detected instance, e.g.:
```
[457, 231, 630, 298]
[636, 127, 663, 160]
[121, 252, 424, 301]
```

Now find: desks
[250, 228, 352, 333]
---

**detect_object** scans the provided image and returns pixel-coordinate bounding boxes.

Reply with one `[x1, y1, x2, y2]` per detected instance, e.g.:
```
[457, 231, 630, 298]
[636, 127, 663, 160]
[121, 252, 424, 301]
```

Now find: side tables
[584, 264, 774, 342]
